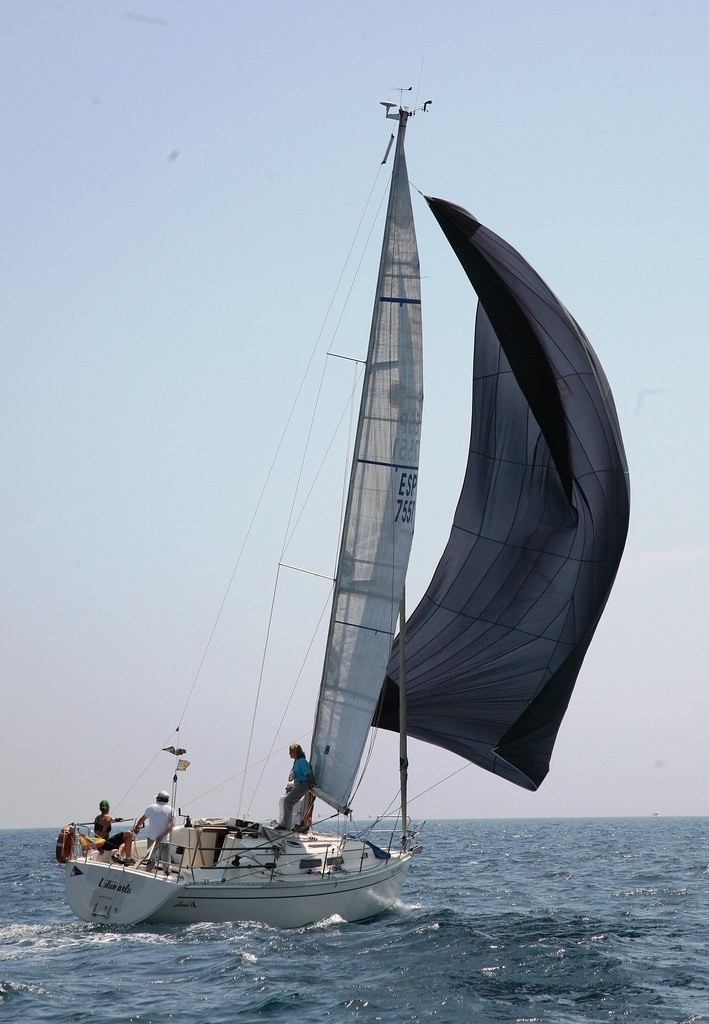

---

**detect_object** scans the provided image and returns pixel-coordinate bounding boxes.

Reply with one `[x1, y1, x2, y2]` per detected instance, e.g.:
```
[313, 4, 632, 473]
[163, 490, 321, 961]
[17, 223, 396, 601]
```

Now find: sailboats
[47, 88, 634, 935]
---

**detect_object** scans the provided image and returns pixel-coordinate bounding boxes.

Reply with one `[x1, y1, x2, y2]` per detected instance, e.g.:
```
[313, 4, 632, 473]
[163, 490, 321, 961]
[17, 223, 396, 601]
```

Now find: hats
[285, 783, 293, 788]
[155, 791, 170, 798]
[100, 800, 109, 809]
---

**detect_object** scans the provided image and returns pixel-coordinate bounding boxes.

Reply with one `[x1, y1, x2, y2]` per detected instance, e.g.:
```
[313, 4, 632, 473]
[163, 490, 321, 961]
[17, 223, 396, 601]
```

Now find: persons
[274, 744, 313, 830]
[134, 791, 176, 876]
[279, 781, 302, 829]
[94, 800, 136, 866]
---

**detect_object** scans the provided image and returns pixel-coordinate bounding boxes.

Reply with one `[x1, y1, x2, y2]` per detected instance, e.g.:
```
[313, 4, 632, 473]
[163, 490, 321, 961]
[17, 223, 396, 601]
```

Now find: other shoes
[274, 826, 286, 830]
[111, 853, 124, 864]
[124, 857, 136, 866]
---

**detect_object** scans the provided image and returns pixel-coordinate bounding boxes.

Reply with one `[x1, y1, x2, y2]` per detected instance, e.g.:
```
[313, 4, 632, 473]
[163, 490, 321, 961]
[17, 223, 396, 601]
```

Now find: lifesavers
[56, 826, 74, 863]
